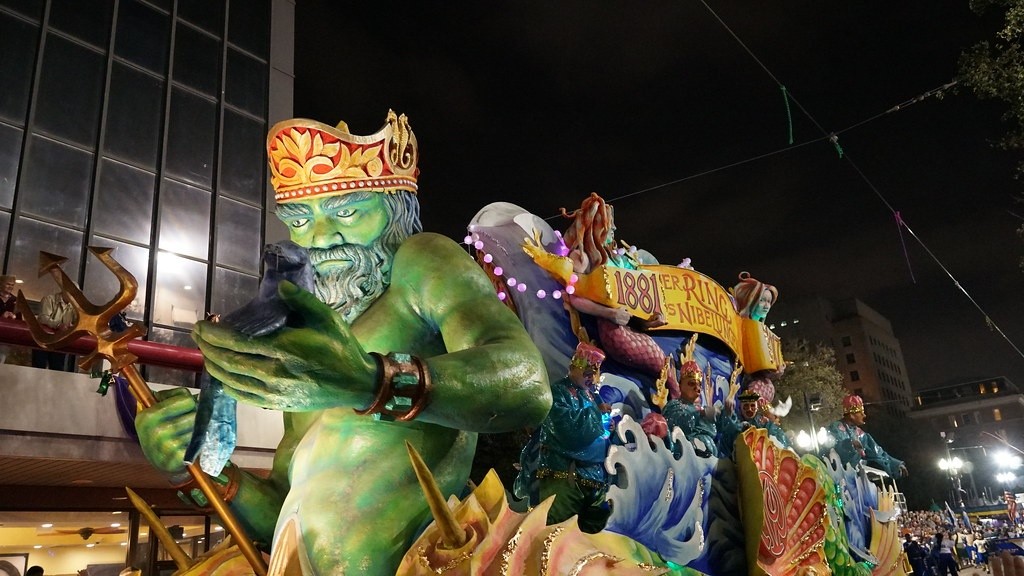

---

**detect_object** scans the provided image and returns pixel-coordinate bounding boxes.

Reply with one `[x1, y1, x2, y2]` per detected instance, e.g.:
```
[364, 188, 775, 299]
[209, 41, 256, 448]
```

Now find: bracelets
[353, 349, 433, 425]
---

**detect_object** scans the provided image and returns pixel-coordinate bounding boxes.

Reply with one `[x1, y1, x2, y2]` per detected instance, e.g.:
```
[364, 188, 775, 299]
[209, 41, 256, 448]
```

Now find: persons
[512, 340, 615, 525]
[135, 108, 552, 576]
[898, 497, 1024, 576]
[559, 193, 679, 401]
[661, 361, 721, 461]
[734, 272, 778, 321]
[737, 389, 788, 448]
[819, 396, 908, 477]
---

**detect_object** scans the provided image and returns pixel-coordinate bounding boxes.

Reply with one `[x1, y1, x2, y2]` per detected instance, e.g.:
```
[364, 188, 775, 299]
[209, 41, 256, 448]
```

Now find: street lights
[939, 457, 968, 511]
[796, 393, 829, 455]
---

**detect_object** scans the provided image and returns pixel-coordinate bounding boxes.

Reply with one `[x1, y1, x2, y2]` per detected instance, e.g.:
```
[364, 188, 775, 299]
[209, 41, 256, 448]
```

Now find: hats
[680, 361, 703, 383]
[576, 340, 605, 368]
[843, 395, 864, 415]
[737, 389, 760, 401]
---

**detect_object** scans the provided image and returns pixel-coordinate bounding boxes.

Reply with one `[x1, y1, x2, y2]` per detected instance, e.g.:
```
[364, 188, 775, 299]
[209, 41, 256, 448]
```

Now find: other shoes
[982, 568, 986, 572]
[987, 570, 991, 574]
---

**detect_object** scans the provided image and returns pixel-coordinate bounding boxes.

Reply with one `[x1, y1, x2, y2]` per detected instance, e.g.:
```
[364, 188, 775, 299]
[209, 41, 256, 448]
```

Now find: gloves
[704, 407, 721, 422]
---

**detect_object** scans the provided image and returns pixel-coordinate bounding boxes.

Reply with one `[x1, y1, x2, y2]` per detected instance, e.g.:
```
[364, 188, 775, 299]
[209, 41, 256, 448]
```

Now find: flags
[944, 501, 956, 528]
